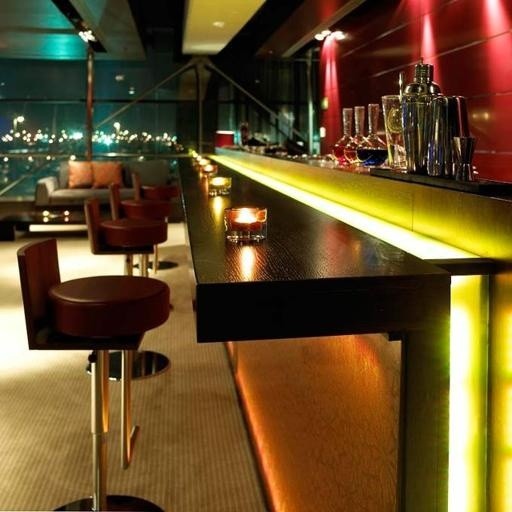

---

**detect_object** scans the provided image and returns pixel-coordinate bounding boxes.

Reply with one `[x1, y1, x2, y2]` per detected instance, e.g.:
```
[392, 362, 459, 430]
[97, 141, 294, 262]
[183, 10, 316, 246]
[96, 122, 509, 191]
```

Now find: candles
[191, 149, 269, 241]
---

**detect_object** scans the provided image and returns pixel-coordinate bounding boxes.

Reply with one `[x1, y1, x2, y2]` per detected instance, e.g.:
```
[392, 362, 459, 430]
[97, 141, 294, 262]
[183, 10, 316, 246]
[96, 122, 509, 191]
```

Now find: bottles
[331, 103, 388, 168]
[402, 62, 442, 174]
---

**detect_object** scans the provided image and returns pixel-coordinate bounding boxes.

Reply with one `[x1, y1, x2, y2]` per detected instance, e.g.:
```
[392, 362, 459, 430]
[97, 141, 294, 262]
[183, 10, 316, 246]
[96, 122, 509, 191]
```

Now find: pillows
[67, 160, 124, 190]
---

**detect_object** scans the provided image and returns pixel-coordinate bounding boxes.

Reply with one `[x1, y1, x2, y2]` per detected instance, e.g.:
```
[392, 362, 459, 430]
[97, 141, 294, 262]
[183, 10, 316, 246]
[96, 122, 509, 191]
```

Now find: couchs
[34, 160, 182, 209]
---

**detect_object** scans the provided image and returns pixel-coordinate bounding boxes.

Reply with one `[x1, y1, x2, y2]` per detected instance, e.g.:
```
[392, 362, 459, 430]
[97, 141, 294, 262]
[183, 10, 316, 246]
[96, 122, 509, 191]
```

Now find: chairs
[84, 171, 178, 381]
[17, 238, 174, 511]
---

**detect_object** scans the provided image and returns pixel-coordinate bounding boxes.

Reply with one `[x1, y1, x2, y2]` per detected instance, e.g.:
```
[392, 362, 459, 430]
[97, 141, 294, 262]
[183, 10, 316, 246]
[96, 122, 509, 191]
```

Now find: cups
[199, 164, 219, 177]
[208, 175, 232, 197]
[451, 135, 476, 182]
[380, 93, 409, 174]
[223, 206, 268, 243]
